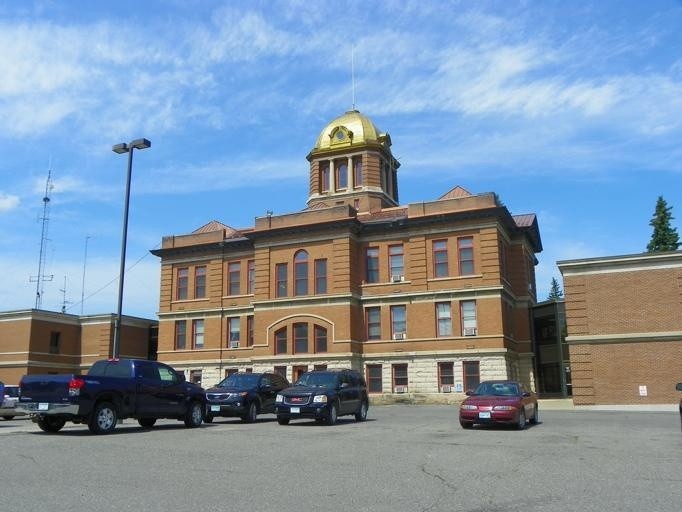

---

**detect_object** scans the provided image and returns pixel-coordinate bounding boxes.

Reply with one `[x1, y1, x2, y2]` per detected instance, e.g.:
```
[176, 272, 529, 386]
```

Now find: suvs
[204, 371, 288, 423]
[276, 368, 370, 425]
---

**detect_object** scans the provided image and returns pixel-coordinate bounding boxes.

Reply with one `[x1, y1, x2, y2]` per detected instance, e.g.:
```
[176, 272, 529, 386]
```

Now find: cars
[459, 379, 538, 430]
[1, 381, 5, 408]
[676, 381, 682, 424]
[2, 384, 32, 420]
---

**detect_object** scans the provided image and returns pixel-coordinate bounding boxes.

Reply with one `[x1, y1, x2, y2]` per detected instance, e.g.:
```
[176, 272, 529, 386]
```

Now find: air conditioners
[396, 386, 404, 392]
[394, 333, 403, 339]
[392, 274, 400, 282]
[231, 341, 238, 348]
[441, 385, 452, 393]
[463, 327, 475, 336]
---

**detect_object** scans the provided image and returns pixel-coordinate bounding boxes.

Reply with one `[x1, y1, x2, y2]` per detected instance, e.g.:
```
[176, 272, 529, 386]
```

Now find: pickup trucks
[14, 356, 207, 432]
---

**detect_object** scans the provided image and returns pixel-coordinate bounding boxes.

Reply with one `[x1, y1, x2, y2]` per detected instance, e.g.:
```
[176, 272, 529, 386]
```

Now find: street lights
[111, 138, 153, 357]
